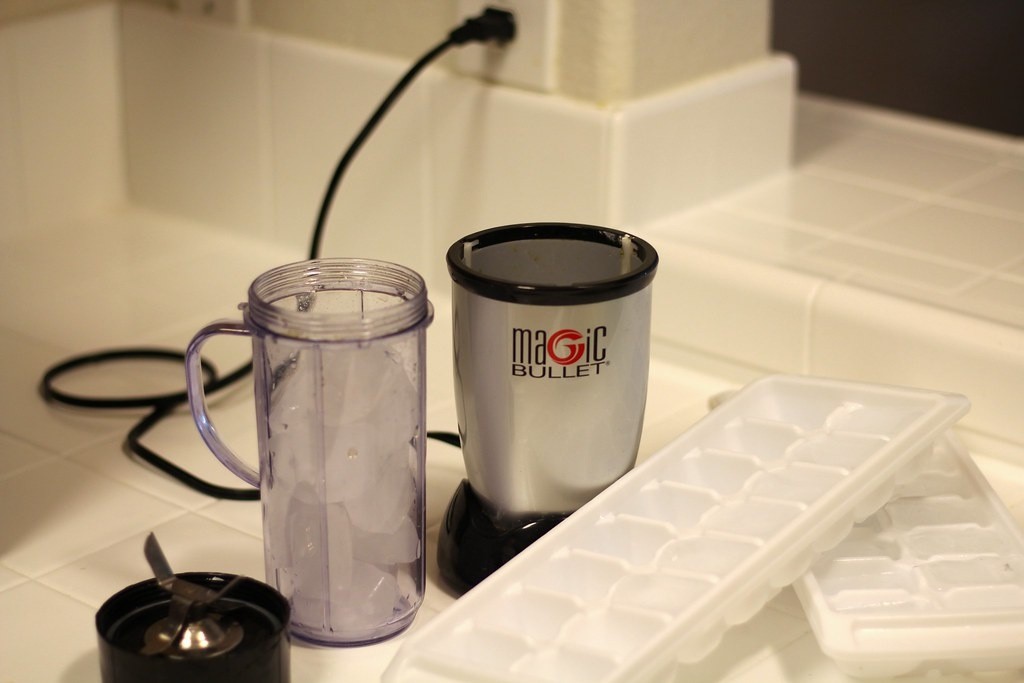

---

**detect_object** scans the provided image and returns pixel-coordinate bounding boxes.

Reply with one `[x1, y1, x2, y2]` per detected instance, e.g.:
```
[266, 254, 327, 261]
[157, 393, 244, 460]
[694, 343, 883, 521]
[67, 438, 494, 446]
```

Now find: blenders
[433, 222, 658, 593]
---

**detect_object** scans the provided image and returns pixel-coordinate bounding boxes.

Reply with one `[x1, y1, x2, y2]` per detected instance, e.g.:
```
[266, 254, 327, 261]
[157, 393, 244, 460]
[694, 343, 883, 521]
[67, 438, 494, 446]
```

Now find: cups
[185, 258, 435, 651]
